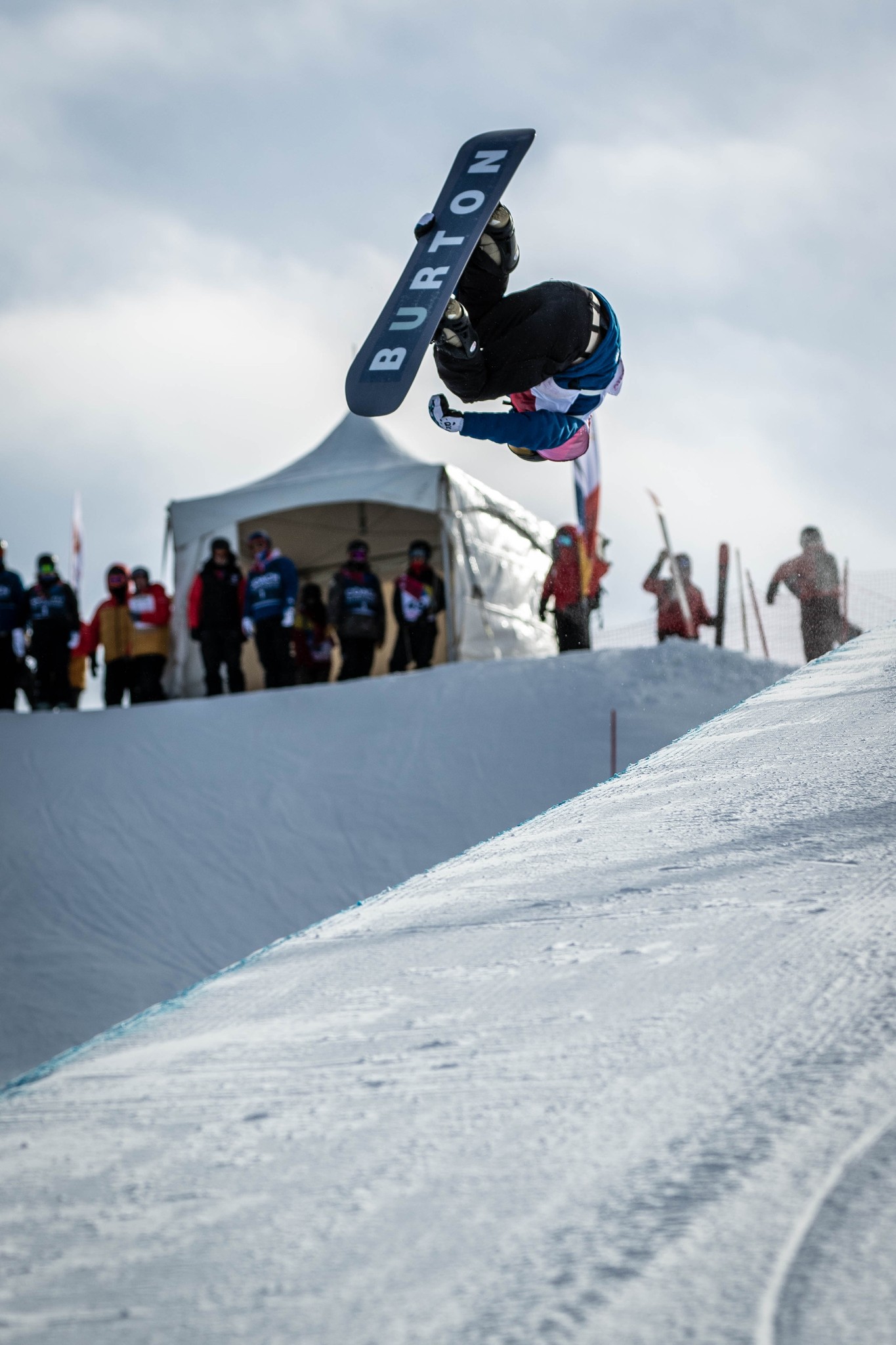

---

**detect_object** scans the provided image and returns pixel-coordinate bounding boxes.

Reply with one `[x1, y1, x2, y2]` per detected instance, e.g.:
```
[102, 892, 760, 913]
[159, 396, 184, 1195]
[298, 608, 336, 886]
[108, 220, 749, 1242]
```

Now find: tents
[167, 410, 559, 702]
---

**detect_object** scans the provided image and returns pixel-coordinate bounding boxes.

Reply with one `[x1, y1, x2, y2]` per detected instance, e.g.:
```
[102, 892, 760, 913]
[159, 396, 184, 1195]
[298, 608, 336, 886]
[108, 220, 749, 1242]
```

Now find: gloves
[429, 395, 466, 434]
[590, 594, 598, 608]
[539, 597, 546, 619]
[282, 608, 297, 629]
[90, 650, 98, 675]
[192, 626, 201, 638]
[67, 632, 80, 648]
[241, 617, 256, 638]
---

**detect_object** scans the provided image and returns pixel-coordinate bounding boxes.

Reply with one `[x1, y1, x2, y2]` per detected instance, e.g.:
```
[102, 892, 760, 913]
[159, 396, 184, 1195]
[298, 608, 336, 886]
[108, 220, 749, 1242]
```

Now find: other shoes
[486, 206, 513, 233]
[442, 302, 467, 328]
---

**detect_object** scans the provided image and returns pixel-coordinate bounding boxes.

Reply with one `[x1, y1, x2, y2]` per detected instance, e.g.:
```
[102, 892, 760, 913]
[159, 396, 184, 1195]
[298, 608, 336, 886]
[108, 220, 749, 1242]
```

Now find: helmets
[556, 526, 579, 557]
[506, 440, 548, 462]
[248, 532, 272, 558]
[108, 567, 128, 599]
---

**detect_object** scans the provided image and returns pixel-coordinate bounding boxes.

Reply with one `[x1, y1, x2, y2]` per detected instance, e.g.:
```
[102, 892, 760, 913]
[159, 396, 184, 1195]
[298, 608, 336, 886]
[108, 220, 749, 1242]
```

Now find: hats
[36, 555, 54, 573]
[406, 537, 433, 562]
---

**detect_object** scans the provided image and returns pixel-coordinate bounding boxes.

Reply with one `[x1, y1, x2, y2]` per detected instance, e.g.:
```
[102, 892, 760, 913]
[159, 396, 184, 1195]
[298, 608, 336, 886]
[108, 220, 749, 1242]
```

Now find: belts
[575, 293, 601, 365]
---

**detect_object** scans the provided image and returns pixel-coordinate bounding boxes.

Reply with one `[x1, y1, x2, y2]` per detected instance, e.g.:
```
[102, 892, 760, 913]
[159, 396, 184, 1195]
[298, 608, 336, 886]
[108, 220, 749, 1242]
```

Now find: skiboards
[650, 493, 731, 651]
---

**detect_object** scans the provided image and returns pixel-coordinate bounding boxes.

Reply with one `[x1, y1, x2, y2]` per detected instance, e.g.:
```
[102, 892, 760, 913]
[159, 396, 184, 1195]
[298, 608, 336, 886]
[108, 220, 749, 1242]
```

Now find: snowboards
[345, 125, 535, 417]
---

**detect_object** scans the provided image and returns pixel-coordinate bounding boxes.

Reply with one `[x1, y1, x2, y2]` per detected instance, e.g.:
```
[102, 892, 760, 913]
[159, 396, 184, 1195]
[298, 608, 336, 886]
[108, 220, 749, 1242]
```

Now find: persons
[326, 539, 387, 682]
[87, 565, 135, 708]
[386, 540, 447, 673]
[187, 539, 250, 696]
[0, 540, 30, 711]
[241, 532, 301, 688]
[642, 551, 718, 641]
[69, 622, 89, 711]
[292, 582, 335, 682]
[124, 569, 172, 706]
[413, 199, 627, 463]
[20, 552, 84, 709]
[767, 528, 861, 662]
[537, 526, 602, 655]
